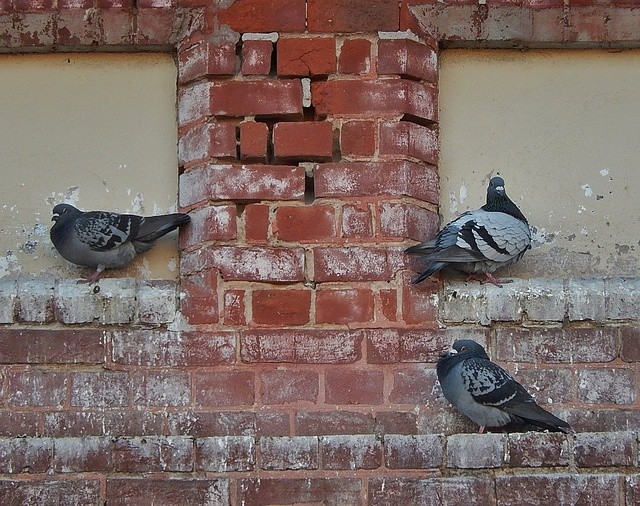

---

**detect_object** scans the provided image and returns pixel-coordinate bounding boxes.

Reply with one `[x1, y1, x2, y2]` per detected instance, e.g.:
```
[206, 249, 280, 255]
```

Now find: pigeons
[435, 338, 571, 437]
[401, 177, 531, 289]
[50, 203, 190, 286]
